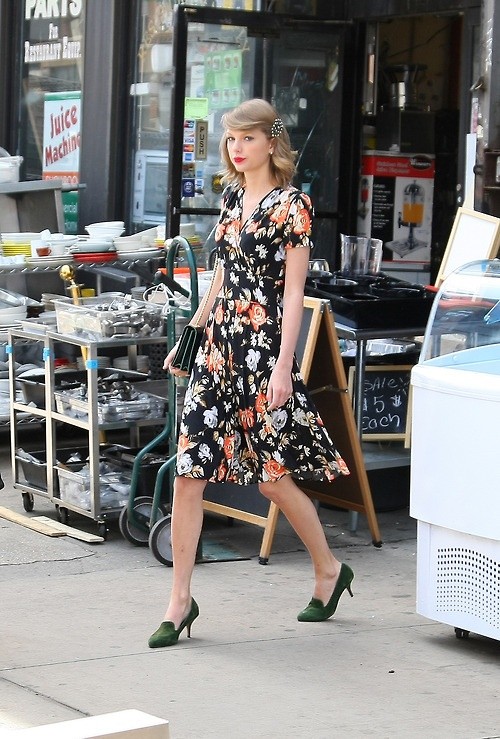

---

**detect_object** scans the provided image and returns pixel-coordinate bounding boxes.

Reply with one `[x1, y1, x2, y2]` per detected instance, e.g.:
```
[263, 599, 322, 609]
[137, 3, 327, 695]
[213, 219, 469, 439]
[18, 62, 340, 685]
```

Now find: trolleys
[118, 235, 201, 564]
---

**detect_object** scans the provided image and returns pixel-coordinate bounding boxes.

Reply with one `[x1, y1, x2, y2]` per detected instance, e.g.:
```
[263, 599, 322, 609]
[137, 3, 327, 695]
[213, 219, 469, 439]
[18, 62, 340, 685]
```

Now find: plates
[154, 235, 203, 248]
[132, 287, 147, 300]
[77, 355, 148, 369]
[0, 232, 159, 268]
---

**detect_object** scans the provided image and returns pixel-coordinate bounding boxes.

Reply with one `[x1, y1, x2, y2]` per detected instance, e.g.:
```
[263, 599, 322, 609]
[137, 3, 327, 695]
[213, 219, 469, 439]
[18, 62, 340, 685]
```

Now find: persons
[149, 98, 354, 648]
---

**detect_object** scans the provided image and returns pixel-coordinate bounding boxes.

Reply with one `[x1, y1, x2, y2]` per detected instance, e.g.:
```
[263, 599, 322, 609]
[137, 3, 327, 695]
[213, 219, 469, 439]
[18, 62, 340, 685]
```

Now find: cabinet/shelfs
[6, 327, 184, 523]
[334, 313, 486, 471]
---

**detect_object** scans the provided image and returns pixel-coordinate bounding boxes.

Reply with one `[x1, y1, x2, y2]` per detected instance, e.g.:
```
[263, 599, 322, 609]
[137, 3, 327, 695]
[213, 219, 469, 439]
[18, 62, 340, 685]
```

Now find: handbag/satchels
[171, 324, 206, 374]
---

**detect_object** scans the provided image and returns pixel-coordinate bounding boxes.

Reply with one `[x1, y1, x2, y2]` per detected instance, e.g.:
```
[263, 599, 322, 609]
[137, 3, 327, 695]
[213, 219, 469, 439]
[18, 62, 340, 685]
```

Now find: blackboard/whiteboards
[202, 297, 372, 528]
[348, 366, 413, 441]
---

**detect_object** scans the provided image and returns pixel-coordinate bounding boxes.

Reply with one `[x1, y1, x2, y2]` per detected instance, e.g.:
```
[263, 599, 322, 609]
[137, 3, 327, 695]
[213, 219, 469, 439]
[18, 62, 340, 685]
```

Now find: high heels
[148, 597, 199, 648]
[297, 563, 354, 622]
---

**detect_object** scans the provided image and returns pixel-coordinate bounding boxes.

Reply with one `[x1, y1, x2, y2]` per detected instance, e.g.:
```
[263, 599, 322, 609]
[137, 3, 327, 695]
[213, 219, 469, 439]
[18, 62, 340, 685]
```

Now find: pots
[312, 277, 359, 294]
[306, 270, 333, 287]
[19, 378, 81, 409]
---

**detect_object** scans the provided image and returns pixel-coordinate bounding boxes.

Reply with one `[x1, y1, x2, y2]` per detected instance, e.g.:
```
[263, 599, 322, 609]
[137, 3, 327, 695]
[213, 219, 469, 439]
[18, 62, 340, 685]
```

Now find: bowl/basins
[0, 304, 27, 323]
[41, 293, 68, 311]
[0, 343, 40, 363]
[77, 221, 142, 251]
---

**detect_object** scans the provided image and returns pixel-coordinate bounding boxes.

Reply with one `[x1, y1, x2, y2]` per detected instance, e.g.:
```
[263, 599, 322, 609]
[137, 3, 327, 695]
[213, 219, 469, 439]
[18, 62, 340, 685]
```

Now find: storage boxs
[303, 273, 442, 329]
[49, 296, 164, 340]
[100, 445, 170, 505]
[53, 462, 132, 510]
[14, 368, 152, 409]
[54, 388, 168, 424]
[357, 156, 435, 263]
[15, 443, 132, 490]
